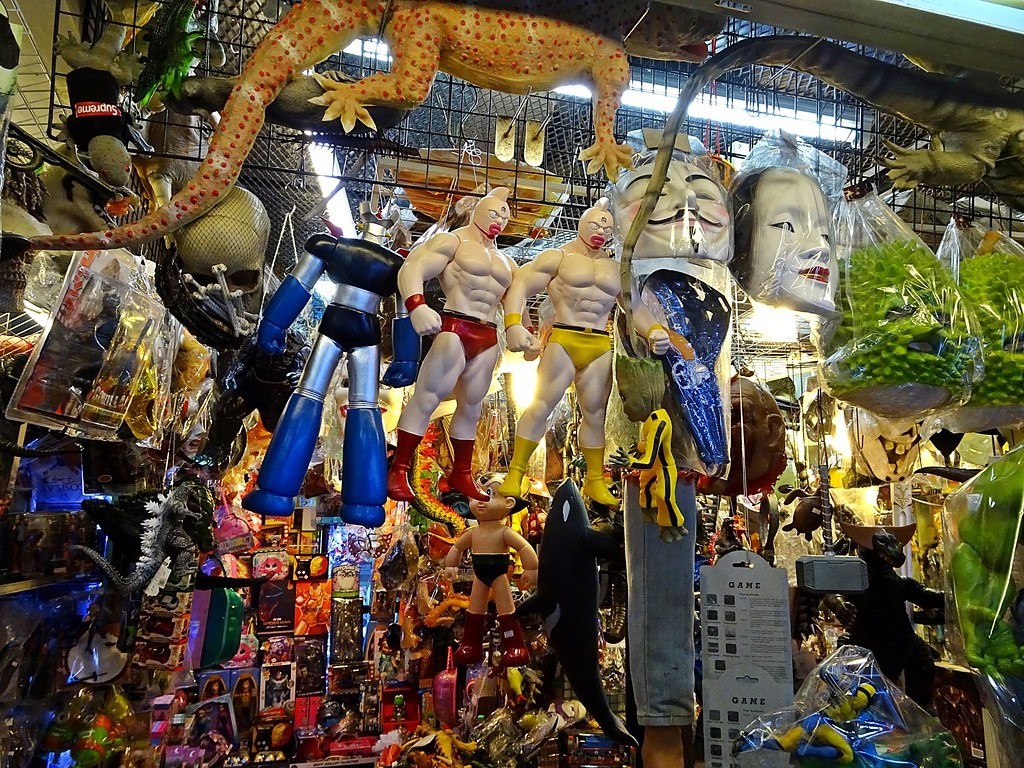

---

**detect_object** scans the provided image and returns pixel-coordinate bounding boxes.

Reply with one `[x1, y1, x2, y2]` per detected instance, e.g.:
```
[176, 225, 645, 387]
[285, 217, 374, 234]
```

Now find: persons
[239, 128, 842, 768]
